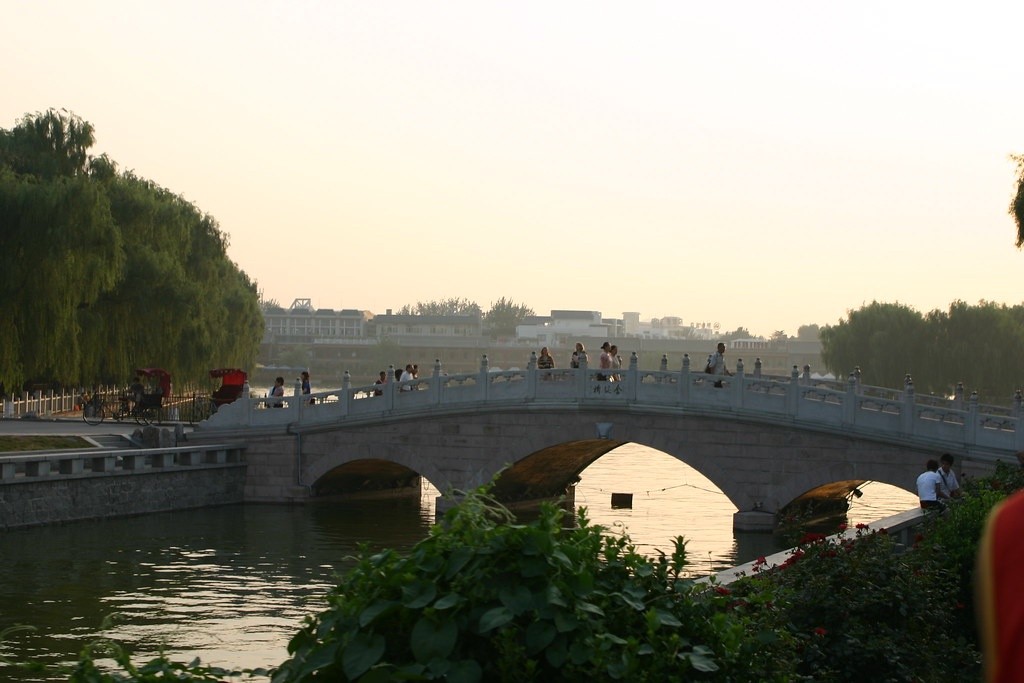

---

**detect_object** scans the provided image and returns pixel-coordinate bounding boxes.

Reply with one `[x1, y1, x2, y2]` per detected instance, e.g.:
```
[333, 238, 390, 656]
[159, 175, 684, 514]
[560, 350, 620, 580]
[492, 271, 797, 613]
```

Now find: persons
[398, 364, 419, 391]
[267, 376, 284, 408]
[130, 377, 144, 394]
[571, 342, 588, 369]
[538, 347, 554, 369]
[375, 371, 386, 396]
[598, 342, 620, 381]
[709, 342, 733, 388]
[915, 453, 961, 510]
[301, 371, 315, 405]
[77, 390, 93, 405]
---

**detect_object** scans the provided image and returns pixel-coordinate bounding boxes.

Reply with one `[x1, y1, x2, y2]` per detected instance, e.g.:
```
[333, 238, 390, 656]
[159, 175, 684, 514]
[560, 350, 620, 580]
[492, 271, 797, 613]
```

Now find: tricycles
[82, 367, 171, 427]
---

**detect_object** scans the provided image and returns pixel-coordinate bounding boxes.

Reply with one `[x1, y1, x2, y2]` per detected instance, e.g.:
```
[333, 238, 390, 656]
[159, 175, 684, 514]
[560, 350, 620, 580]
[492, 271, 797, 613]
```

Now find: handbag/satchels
[950, 490, 960, 499]
[705, 367, 711, 373]
